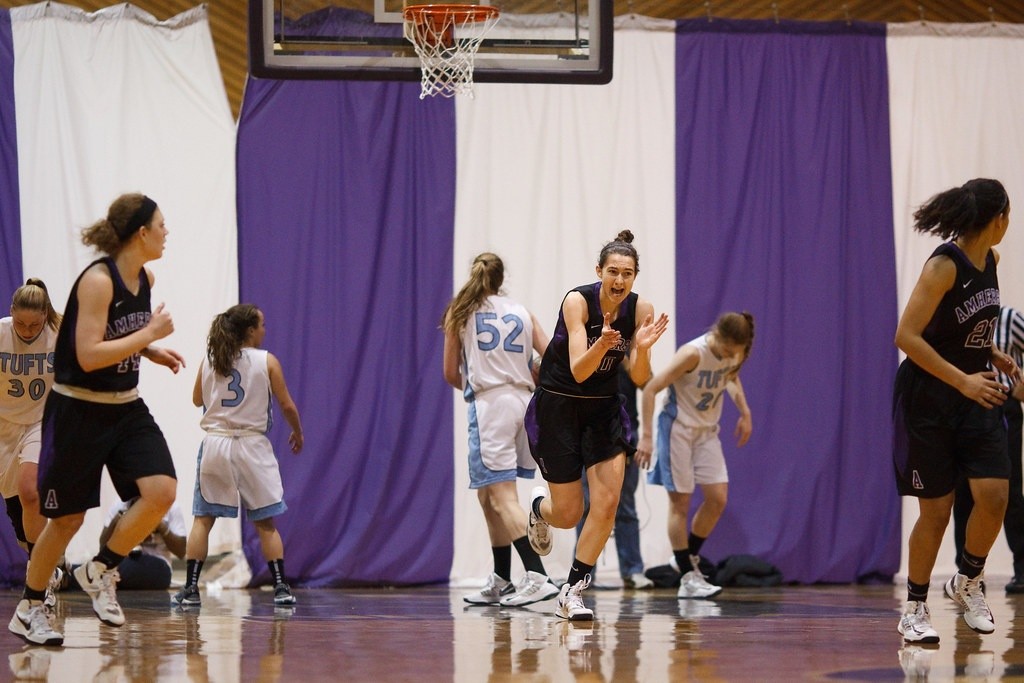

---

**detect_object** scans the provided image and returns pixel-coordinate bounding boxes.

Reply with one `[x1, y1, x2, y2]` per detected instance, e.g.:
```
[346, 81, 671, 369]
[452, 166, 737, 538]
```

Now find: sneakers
[465, 606, 500, 618]
[896, 647, 940, 676]
[463, 571, 517, 605]
[966, 650, 994, 679]
[677, 571, 723, 599]
[8, 648, 52, 680]
[274, 583, 296, 605]
[73, 560, 125, 628]
[528, 486, 554, 556]
[8, 603, 64, 646]
[172, 584, 201, 603]
[175, 606, 201, 619]
[555, 573, 594, 620]
[945, 570, 995, 634]
[43, 606, 55, 624]
[678, 599, 720, 618]
[1005, 576, 1024, 593]
[41, 567, 63, 606]
[562, 623, 594, 650]
[498, 572, 560, 607]
[669, 552, 706, 583]
[898, 598, 940, 645]
[274, 607, 297, 621]
[623, 572, 654, 589]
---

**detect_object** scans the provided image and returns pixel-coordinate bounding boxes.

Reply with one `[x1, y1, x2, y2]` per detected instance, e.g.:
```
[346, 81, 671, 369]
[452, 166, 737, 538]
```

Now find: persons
[9, 193, 187, 646]
[443, 252, 560, 607]
[172, 305, 304, 603]
[1, 278, 63, 607]
[634, 314, 754, 598]
[576, 430, 654, 588]
[953, 304, 1024, 592]
[528, 230, 669, 621]
[892, 178, 1024, 640]
[54, 498, 187, 593]
